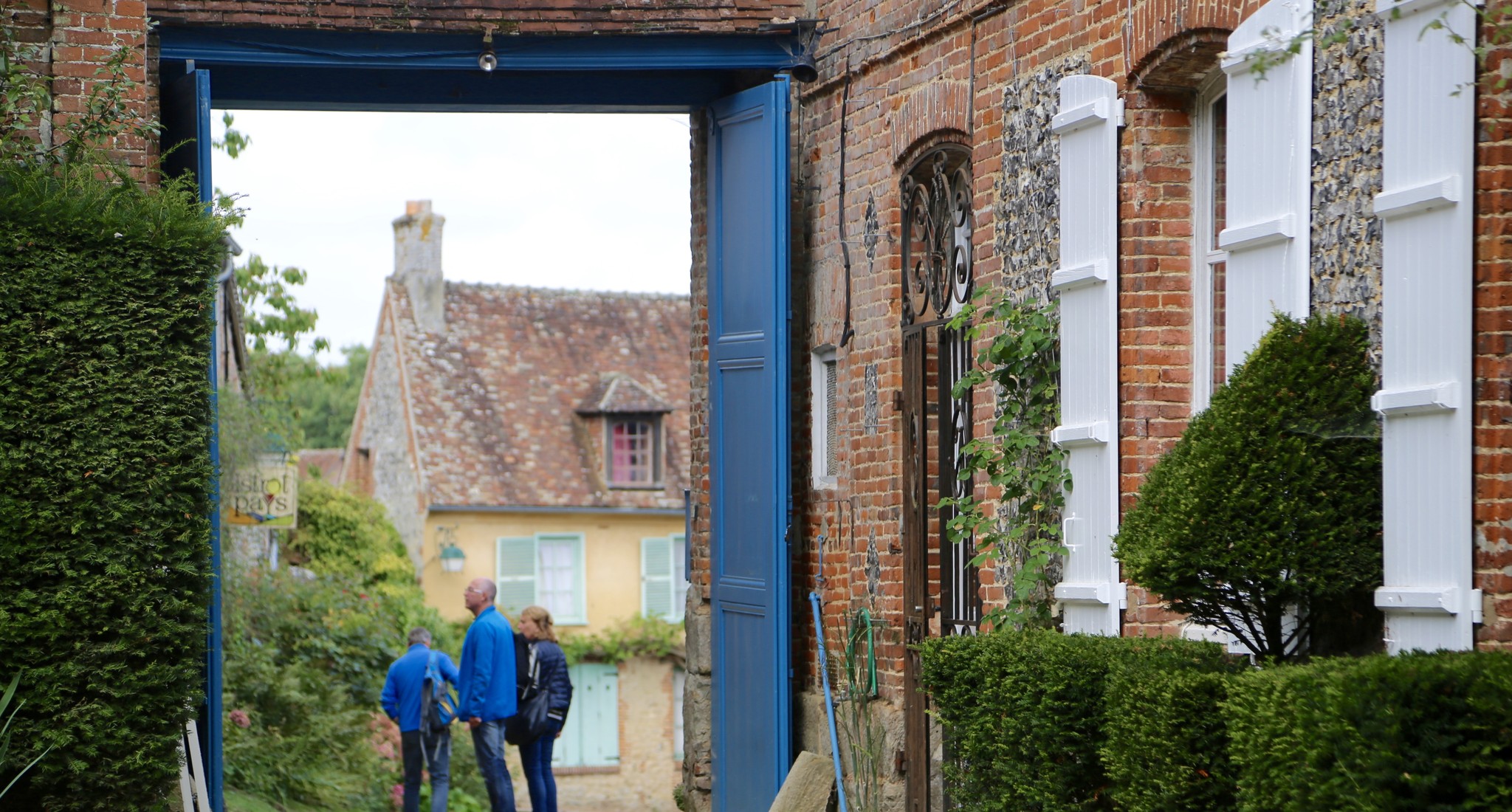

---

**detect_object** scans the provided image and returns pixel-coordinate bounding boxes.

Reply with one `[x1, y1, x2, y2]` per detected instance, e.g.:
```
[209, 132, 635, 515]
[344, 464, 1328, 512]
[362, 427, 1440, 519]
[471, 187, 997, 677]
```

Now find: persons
[460, 577, 520, 812]
[376, 627, 466, 812]
[516, 605, 572, 812]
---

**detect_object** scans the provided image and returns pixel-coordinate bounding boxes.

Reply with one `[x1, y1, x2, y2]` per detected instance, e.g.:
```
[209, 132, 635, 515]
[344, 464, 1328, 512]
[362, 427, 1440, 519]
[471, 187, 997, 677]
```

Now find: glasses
[467, 587, 485, 594]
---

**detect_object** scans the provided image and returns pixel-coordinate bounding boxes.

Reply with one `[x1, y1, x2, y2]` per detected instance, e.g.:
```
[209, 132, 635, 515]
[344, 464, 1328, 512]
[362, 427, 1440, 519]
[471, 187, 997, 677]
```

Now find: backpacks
[418, 651, 461, 737]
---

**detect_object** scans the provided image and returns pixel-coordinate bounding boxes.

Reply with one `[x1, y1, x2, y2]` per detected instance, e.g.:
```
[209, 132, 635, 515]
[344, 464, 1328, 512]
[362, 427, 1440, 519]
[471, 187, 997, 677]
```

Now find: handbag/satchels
[503, 690, 551, 743]
